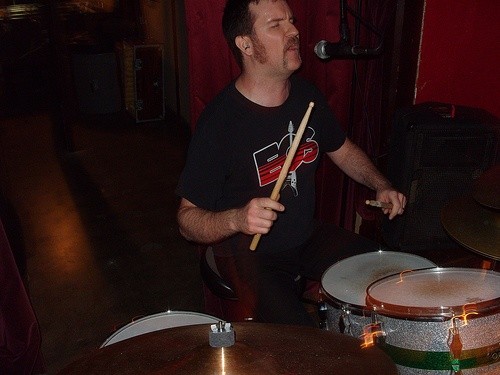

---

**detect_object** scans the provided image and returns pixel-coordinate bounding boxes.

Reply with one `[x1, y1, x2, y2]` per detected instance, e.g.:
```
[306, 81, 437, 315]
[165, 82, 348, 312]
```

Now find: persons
[172, 0, 407, 329]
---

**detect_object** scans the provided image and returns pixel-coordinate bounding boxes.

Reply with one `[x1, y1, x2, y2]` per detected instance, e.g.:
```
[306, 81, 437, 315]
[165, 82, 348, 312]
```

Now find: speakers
[378, 101, 500, 255]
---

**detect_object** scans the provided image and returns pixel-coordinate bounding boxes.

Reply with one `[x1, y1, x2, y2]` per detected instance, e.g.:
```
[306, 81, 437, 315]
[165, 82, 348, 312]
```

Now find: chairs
[198, 241, 323, 323]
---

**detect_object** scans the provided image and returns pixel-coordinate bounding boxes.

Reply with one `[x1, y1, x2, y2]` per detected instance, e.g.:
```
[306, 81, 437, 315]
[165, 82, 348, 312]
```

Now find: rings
[402, 208, 405, 210]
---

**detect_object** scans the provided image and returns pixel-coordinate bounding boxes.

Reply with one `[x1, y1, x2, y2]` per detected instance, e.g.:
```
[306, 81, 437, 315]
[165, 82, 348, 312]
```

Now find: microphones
[314, 40, 378, 59]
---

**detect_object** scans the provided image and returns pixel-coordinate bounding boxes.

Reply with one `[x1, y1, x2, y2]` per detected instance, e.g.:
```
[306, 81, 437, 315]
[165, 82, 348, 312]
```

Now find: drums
[319, 251, 440, 339]
[98, 310, 227, 349]
[365, 267, 500, 375]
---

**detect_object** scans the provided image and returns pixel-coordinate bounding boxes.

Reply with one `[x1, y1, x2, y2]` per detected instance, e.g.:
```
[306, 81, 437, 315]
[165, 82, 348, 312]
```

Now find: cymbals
[54, 322, 398, 375]
[440, 164, 500, 261]
